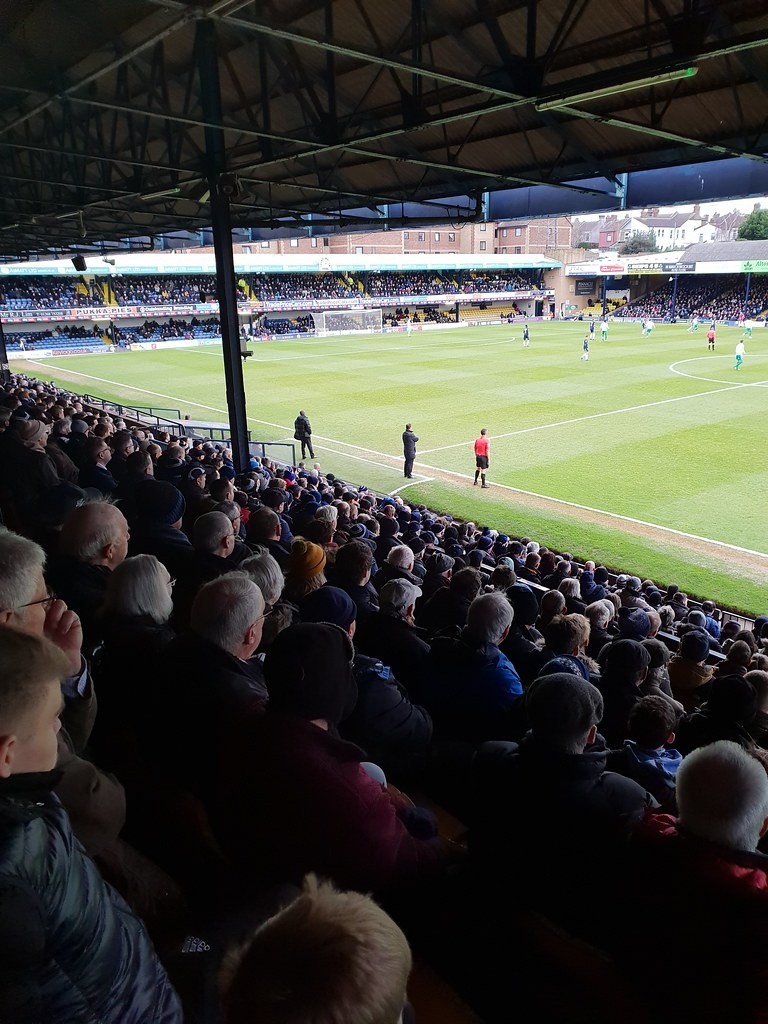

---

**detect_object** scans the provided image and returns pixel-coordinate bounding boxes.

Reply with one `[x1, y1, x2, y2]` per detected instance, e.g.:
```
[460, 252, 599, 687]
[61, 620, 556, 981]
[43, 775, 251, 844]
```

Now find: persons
[0, 367, 768, 1024]
[1, 272, 765, 367]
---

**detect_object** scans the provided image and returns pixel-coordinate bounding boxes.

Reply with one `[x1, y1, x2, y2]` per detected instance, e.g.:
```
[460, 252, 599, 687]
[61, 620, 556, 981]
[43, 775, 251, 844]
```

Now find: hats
[378, 578, 424, 610]
[536, 654, 588, 681]
[300, 586, 357, 630]
[8, 405, 88, 441]
[707, 674, 757, 723]
[581, 563, 766, 639]
[178, 440, 235, 483]
[680, 631, 709, 662]
[283, 470, 372, 541]
[286, 540, 327, 577]
[260, 621, 358, 723]
[377, 496, 518, 578]
[526, 672, 604, 732]
[603, 639, 651, 673]
[135, 479, 186, 525]
[504, 585, 539, 625]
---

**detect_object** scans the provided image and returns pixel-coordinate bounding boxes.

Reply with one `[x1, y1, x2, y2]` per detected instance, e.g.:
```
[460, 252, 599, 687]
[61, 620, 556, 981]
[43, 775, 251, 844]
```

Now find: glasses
[13, 584, 55, 611]
[231, 514, 244, 521]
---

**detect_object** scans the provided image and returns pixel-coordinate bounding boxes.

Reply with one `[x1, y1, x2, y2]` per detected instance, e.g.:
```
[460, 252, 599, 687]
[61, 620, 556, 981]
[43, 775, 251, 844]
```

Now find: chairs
[1, 270, 766, 1024]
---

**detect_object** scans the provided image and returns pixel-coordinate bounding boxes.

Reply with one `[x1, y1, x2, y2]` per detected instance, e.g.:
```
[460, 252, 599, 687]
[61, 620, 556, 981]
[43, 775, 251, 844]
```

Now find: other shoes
[407, 475, 414, 479]
[474, 482, 479, 485]
[482, 485, 489, 488]
[404, 475, 407, 477]
[313, 456, 318, 458]
[303, 457, 307, 459]
